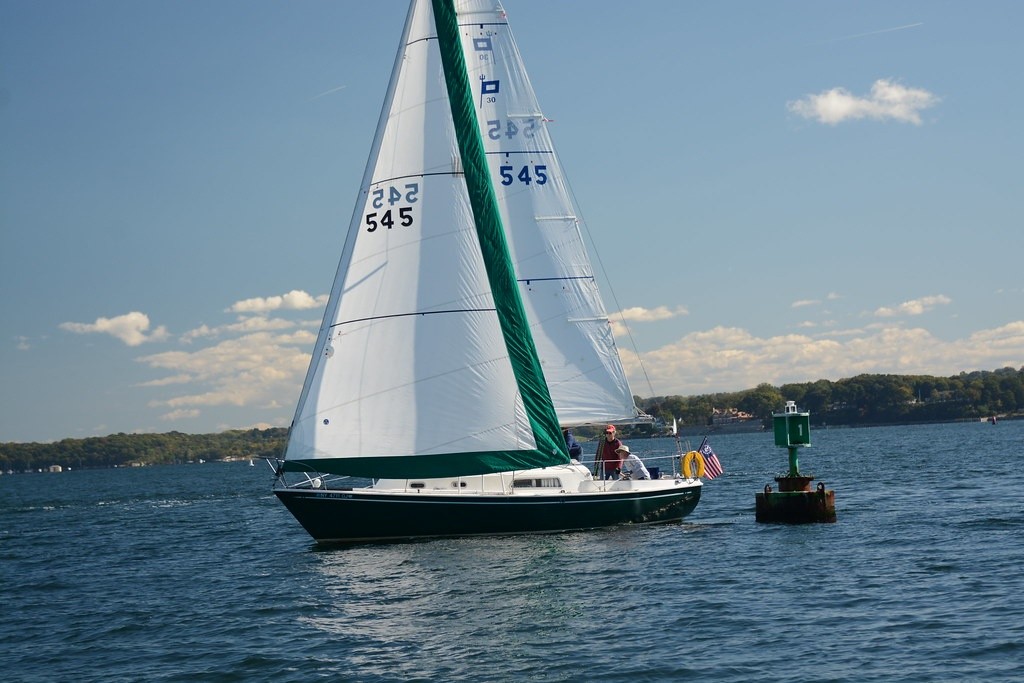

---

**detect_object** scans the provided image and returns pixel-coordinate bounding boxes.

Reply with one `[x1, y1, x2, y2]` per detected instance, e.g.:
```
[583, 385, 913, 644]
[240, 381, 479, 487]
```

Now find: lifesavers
[682, 450, 705, 480]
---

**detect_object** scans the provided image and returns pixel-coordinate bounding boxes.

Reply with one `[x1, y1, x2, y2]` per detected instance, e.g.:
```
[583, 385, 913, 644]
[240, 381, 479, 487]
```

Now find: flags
[699, 441, 723, 480]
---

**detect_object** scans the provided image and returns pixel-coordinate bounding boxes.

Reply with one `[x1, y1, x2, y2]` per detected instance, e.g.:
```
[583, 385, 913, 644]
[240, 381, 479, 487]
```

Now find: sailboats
[250, 459, 255, 467]
[251, 0, 704, 545]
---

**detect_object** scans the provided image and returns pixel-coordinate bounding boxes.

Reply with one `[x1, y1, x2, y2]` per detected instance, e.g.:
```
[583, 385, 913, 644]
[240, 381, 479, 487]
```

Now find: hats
[606, 425, 617, 433]
[614, 445, 630, 455]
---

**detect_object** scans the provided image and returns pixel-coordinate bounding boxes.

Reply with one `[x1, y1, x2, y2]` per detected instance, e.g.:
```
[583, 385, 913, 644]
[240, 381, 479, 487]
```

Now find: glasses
[606, 431, 612, 434]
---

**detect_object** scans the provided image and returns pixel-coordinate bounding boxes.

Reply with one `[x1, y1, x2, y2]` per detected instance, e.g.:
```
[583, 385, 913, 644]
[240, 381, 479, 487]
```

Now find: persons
[615, 445, 651, 480]
[595, 425, 622, 480]
[561, 428, 583, 463]
